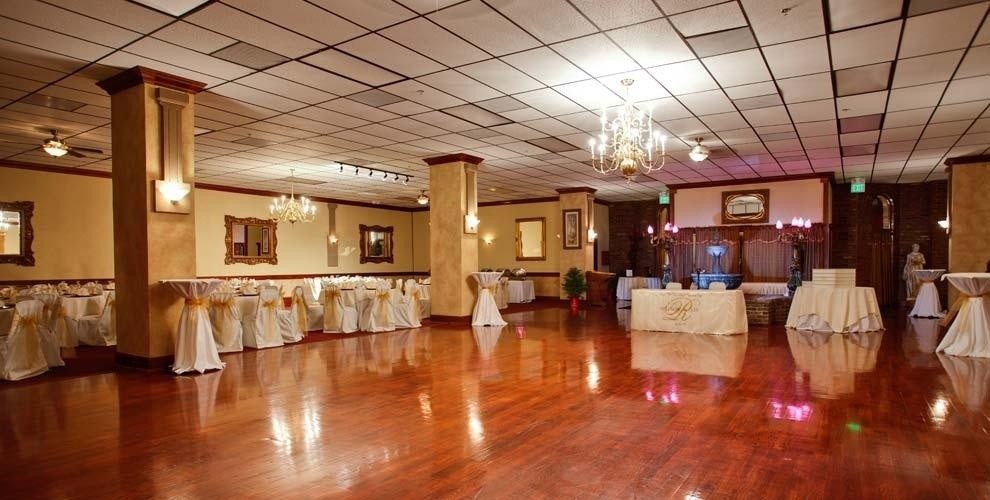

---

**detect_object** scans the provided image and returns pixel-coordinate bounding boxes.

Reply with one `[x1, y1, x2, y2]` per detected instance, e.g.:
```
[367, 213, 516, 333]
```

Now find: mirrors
[513, 216, 548, 262]
[0, 199, 37, 267]
[222, 213, 280, 266]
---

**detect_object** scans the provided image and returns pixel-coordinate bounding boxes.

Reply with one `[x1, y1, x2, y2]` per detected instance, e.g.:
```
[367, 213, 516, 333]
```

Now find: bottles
[226, 277, 255, 287]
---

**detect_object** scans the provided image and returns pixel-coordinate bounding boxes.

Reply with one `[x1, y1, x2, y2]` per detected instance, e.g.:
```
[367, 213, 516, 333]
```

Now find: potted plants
[562, 266, 589, 309]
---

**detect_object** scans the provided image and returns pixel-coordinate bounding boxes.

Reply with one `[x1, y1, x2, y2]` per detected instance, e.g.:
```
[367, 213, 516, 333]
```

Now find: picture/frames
[358, 223, 394, 265]
[561, 209, 583, 250]
[261, 226, 270, 254]
[720, 188, 771, 225]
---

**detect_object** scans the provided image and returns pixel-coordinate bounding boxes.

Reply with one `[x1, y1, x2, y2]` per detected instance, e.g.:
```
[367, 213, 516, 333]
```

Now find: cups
[626, 270, 632, 277]
[0, 286, 14, 308]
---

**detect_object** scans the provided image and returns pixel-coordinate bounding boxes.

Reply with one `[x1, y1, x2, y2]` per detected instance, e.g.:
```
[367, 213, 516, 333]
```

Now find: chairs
[496, 277, 510, 309]
[396, 280, 421, 328]
[419, 285, 430, 319]
[324, 285, 359, 334]
[244, 289, 285, 349]
[1, 300, 49, 380]
[5, 282, 115, 348]
[208, 292, 244, 353]
[708, 280, 726, 290]
[664, 282, 683, 289]
[359, 282, 396, 332]
[280, 286, 308, 343]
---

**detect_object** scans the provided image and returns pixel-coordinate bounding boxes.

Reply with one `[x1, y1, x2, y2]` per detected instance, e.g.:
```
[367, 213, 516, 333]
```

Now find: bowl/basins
[691, 273, 745, 290]
[705, 245, 728, 257]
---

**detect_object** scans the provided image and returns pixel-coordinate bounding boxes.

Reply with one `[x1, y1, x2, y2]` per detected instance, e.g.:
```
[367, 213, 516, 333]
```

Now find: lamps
[464, 211, 482, 235]
[586, 75, 669, 184]
[152, 177, 195, 214]
[41, 128, 71, 160]
[775, 215, 816, 271]
[648, 221, 682, 267]
[339, 163, 410, 187]
[269, 167, 318, 223]
[686, 133, 711, 162]
[417, 188, 431, 207]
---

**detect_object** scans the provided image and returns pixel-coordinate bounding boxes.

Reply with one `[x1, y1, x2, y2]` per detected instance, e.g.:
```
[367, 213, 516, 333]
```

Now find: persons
[902, 244, 927, 298]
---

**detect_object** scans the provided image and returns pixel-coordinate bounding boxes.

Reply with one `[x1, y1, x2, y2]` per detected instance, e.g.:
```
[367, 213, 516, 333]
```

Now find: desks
[616, 276, 664, 300]
[908, 267, 950, 318]
[629, 332, 750, 378]
[933, 272, 990, 358]
[785, 329, 882, 399]
[419, 278, 430, 298]
[585, 269, 616, 307]
[212, 276, 404, 305]
[629, 286, 750, 336]
[784, 283, 889, 334]
[936, 352, 990, 409]
[911, 319, 939, 355]
[509, 280, 535, 303]
